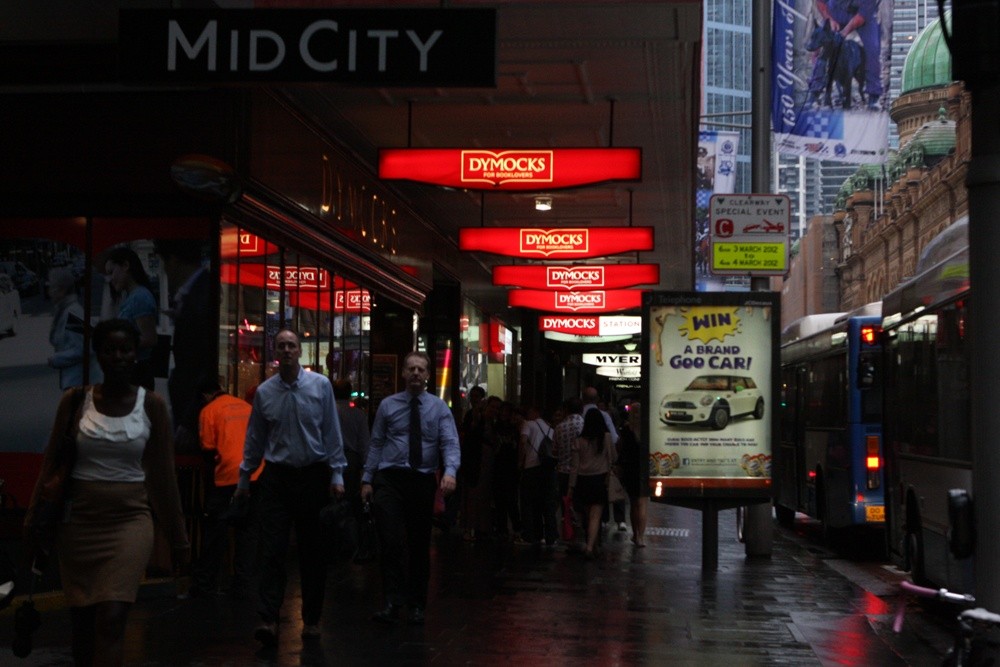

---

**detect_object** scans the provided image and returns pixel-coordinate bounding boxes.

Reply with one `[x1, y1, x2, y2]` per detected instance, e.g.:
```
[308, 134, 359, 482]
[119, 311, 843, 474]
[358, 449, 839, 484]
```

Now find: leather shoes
[254, 621, 279, 641]
[407, 607, 425, 624]
[371, 602, 401, 627]
[301, 622, 321, 638]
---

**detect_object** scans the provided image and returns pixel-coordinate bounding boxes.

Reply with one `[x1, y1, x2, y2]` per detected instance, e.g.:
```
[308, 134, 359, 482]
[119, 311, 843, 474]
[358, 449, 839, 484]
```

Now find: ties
[408, 394, 423, 470]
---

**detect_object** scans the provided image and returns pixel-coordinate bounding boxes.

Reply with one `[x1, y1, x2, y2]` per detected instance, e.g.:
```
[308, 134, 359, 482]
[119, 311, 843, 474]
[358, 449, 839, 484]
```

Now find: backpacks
[527, 419, 553, 458]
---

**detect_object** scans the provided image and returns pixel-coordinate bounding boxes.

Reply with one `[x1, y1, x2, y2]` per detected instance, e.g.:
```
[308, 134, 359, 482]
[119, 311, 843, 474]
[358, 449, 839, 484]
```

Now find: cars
[657, 374, 766, 429]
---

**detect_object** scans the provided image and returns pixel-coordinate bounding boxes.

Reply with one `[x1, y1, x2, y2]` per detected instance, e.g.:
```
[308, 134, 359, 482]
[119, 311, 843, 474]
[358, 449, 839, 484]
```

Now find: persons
[358, 351, 464, 624]
[190, 374, 267, 587]
[445, 374, 650, 557]
[232, 328, 351, 643]
[20, 313, 191, 665]
[804, 0, 887, 109]
[696, 147, 713, 190]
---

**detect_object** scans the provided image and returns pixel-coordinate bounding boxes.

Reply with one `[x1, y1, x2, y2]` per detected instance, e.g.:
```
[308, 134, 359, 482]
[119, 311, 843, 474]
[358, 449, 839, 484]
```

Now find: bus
[776, 299, 885, 525]
[878, 215, 983, 604]
